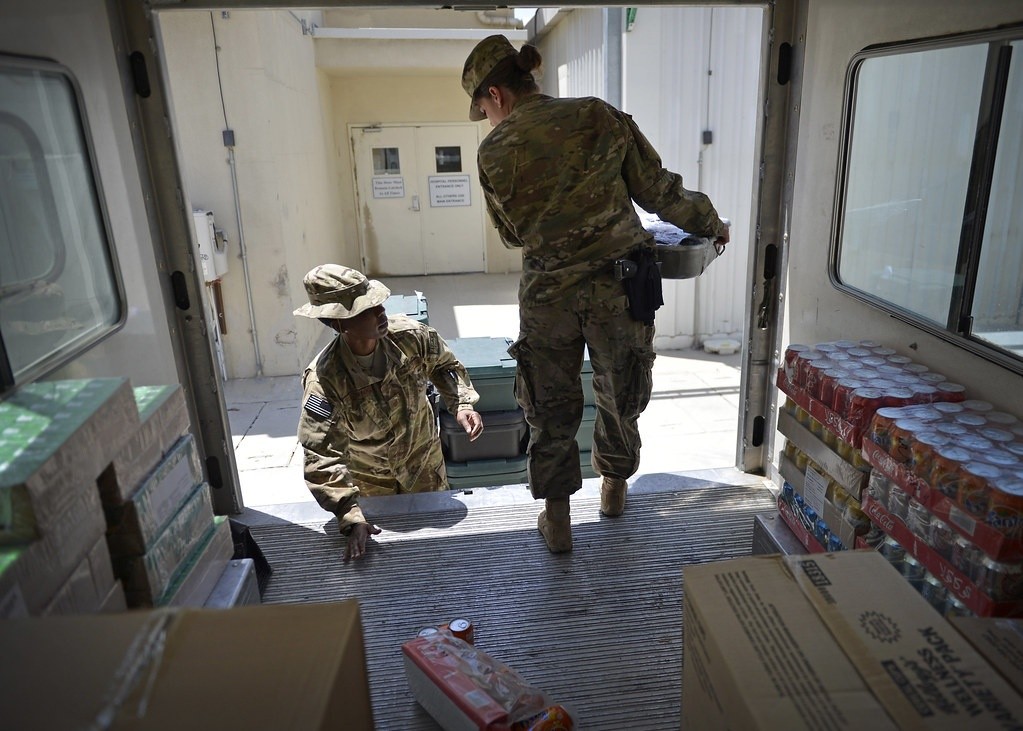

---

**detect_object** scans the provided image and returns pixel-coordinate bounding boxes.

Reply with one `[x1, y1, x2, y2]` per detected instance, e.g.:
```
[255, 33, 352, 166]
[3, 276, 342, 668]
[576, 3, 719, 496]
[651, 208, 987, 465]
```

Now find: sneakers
[537, 509, 574, 555]
[600, 477, 628, 514]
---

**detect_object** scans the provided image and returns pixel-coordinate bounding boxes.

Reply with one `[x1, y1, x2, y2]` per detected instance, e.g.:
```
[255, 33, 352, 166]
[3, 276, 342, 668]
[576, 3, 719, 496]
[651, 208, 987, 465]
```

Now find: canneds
[416, 617, 474, 647]
[513, 692, 575, 731]
[782, 340, 1023, 621]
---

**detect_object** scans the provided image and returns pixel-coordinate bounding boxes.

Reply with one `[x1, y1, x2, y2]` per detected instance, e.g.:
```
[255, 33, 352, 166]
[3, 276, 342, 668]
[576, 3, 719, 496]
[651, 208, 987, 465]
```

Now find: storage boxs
[952, 616, 1023, 697]
[387, 216, 729, 489]
[1, 376, 267, 610]
[680, 550, 1023, 731]
[1, 596, 378, 731]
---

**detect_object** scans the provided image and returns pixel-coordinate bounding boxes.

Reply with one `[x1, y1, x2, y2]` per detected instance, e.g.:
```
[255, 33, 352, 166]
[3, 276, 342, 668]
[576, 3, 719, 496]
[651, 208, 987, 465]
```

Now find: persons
[293, 263, 483, 562]
[461, 34, 730, 553]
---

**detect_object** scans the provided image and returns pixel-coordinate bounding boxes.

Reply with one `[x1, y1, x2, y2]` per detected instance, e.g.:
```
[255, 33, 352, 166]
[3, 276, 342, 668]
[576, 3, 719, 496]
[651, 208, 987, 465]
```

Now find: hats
[461, 34, 542, 122]
[293, 263, 392, 319]
